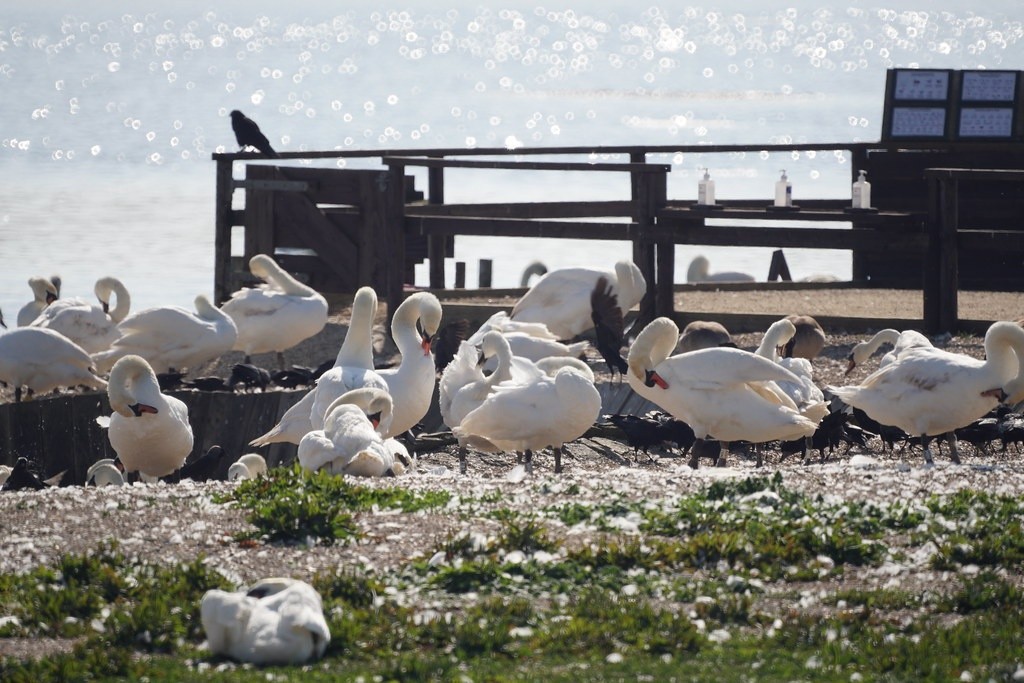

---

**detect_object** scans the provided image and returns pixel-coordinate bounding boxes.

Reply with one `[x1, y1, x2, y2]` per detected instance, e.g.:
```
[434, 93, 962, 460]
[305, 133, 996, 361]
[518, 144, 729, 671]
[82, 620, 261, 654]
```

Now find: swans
[0, 254, 1024, 494]
